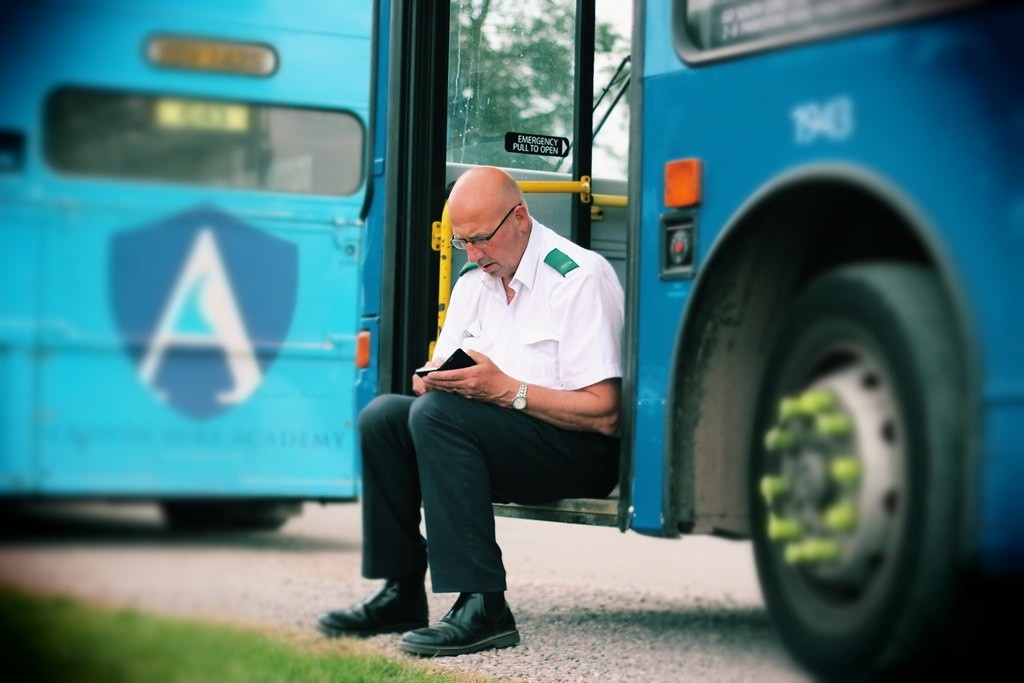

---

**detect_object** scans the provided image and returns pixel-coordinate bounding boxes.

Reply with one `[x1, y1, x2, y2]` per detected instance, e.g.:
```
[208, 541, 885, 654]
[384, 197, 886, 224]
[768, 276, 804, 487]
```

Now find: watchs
[509, 380, 528, 410]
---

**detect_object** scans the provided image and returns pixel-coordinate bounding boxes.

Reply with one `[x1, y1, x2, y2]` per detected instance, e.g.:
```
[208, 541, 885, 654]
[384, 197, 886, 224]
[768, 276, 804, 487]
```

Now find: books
[415, 347, 477, 379]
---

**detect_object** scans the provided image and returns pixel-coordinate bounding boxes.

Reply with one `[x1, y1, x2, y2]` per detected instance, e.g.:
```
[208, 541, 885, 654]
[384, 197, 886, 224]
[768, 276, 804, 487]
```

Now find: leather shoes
[399, 591, 520, 656]
[318, 578, 429, 640]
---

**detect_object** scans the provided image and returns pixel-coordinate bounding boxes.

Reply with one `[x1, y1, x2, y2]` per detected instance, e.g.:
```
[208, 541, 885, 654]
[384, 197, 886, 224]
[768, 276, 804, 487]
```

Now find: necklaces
[506, 292, 514, 297]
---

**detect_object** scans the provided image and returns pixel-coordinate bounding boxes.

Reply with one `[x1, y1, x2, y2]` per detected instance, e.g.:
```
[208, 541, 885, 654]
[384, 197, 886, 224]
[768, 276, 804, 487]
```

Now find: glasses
[452, 203, 522, 250]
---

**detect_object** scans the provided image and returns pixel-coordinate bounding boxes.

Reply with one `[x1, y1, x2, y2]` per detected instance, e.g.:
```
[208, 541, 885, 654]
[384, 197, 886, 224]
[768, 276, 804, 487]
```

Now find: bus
[350, 0, 1024, 682]
[0, 2, 379, 546]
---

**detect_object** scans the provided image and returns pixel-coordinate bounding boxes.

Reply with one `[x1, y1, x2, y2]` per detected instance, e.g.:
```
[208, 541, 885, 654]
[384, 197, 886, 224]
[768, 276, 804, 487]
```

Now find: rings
[452, 386, 458, 393]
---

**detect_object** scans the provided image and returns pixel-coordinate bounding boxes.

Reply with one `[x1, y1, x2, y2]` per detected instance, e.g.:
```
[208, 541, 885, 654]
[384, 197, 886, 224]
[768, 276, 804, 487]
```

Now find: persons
[313, 165, 625, 658]
